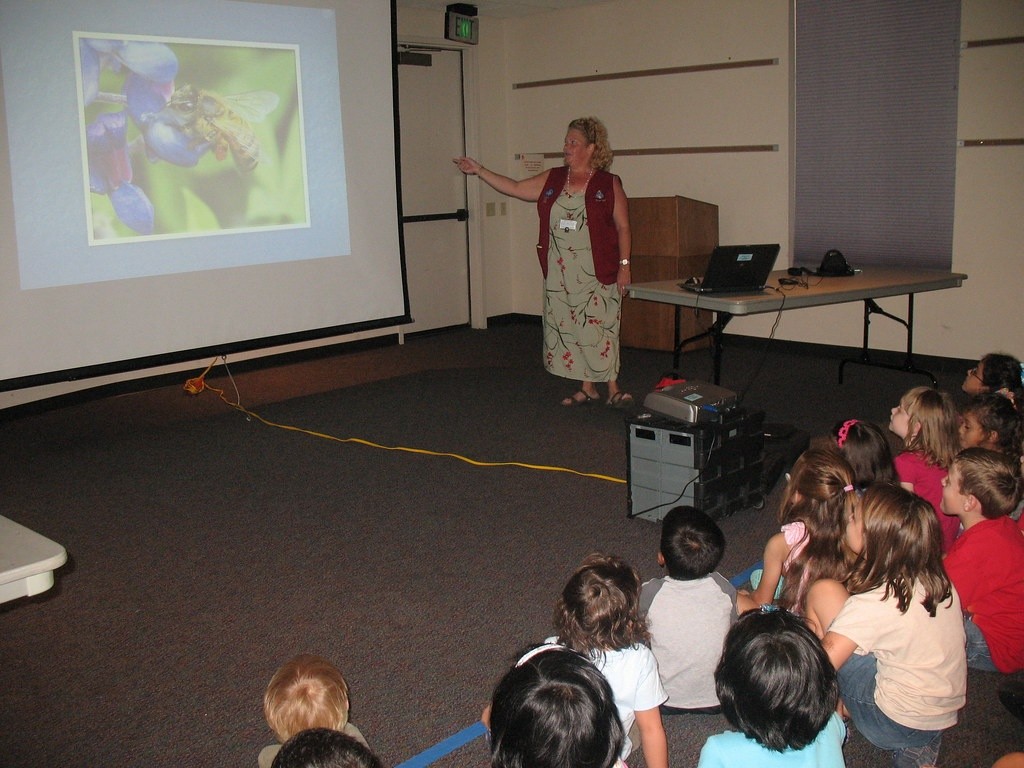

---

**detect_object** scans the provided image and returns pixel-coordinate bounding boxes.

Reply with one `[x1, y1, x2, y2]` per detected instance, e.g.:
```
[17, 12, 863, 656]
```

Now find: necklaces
[567, 167, 593, 196]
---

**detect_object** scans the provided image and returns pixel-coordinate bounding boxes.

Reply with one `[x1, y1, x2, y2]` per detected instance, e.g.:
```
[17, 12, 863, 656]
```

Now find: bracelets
[619, 266, 630, 271]
[477, 166, 485, 179]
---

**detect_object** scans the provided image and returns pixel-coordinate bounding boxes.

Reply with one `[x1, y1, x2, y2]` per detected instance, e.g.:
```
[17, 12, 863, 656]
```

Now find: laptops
[678, 243, 781, 294]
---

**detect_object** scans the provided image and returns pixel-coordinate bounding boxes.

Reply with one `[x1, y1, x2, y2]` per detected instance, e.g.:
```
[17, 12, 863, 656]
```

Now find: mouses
[684, 277, 700, 284]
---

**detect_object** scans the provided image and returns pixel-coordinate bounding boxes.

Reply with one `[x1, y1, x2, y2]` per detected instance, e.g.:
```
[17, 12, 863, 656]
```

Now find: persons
[634, 505, 740, 715]
[544, 554, 670, 768]
[737, 352, 1024, 673]
[453, 116, 636, 409]
[254, 657, 383, 768]
[991, 751, 1024, 768]
[697, 606, 848, 768]
[483, 642, 626, 768]
[806, 485, 968, 768]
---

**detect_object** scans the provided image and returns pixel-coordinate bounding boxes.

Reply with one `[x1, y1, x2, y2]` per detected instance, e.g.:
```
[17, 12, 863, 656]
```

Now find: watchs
[619, 259, 631, 265]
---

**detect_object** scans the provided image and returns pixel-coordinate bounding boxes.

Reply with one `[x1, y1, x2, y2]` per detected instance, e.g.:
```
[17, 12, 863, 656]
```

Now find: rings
[622, 286, 626, 290]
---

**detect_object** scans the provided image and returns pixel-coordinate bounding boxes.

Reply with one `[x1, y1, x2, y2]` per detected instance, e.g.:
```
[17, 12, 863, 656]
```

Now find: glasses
[971, 367, 985, 383]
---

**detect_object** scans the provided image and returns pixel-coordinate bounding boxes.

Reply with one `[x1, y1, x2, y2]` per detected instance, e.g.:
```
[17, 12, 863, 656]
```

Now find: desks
[615, 264, 969, 390]
[0, 514, 68, 603]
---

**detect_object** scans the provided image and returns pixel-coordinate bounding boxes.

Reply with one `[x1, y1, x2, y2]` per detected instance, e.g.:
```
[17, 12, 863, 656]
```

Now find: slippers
[560, 389, 603, 408]
[603, 392, 634, 409]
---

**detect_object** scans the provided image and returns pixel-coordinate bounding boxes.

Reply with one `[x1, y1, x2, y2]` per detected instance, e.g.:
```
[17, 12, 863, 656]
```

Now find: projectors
[643, 378, 738, 426]
